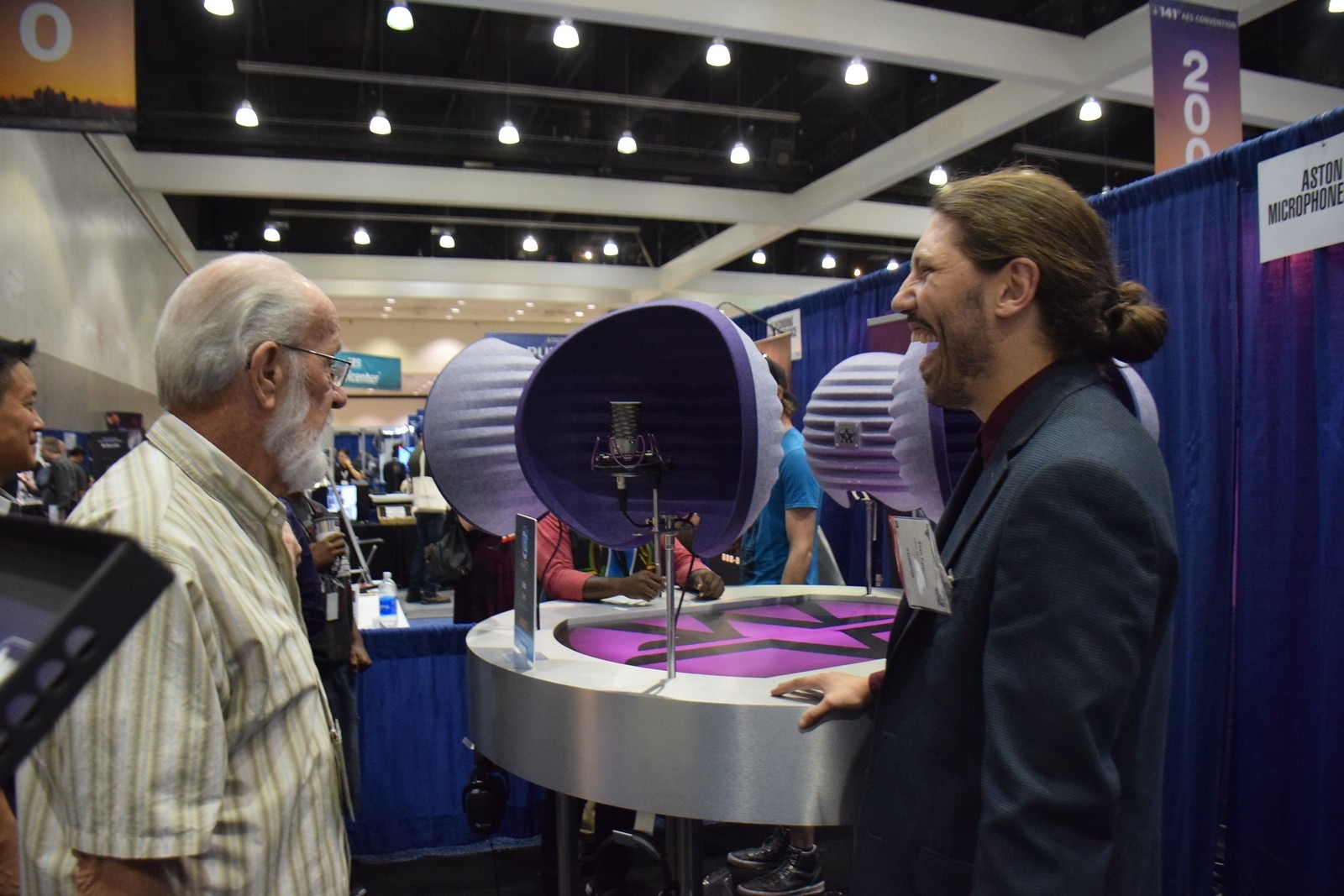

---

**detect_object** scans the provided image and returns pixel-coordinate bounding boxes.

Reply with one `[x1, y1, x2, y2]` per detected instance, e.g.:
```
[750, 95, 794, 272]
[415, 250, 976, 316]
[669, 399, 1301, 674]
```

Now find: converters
[460, 762, 511, 839]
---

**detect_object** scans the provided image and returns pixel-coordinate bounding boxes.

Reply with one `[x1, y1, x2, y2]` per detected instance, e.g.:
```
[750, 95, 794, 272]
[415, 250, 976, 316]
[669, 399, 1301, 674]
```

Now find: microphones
[589, 400, 672, 511]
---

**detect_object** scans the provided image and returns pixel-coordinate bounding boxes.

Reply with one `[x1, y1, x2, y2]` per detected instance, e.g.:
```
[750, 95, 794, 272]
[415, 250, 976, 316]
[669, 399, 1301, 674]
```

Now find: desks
[461, 581, 906, 896]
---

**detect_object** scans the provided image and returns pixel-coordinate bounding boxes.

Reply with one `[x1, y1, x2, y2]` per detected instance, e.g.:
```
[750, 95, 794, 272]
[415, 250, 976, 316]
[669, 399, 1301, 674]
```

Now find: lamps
[203, 0, 1105, 270]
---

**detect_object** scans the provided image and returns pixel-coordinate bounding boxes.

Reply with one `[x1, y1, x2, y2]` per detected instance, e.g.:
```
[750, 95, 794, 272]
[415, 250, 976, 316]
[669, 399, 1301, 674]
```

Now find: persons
[771, 164, 1186, 895]
[0, 250, 829, 896]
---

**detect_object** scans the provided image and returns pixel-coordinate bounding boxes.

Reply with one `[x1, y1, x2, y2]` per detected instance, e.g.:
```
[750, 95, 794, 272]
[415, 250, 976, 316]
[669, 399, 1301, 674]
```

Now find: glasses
[245, 342, 352, 386]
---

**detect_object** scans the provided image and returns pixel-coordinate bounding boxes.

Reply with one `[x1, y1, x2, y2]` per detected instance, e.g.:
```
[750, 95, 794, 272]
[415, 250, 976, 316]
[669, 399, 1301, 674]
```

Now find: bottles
[378, 570, 398, 628]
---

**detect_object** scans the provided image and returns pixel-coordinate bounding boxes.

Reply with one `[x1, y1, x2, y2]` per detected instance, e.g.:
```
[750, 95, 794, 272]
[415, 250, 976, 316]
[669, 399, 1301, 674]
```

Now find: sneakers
[737, 844, 825, 896]
[728, 826, 791, 870]
